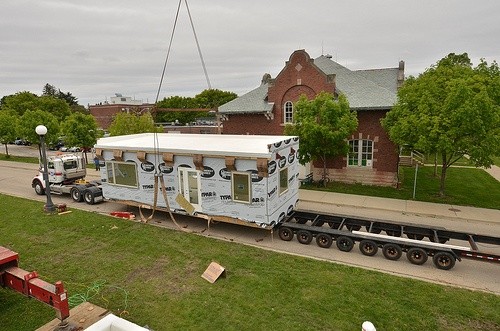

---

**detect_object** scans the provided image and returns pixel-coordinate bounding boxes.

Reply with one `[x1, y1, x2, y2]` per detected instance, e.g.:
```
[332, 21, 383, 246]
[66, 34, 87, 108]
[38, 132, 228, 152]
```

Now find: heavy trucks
[32, 131, 500, 270]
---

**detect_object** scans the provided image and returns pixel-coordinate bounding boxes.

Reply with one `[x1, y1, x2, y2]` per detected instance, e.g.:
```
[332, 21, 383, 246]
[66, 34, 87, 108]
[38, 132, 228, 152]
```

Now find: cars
[14, 137, 82, 152]
